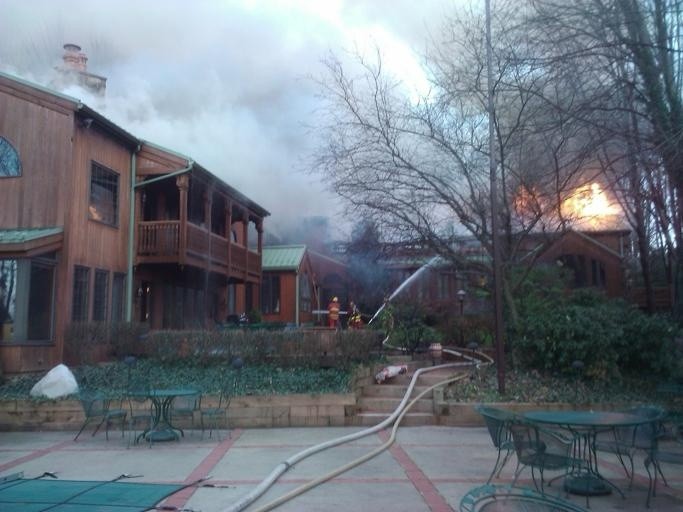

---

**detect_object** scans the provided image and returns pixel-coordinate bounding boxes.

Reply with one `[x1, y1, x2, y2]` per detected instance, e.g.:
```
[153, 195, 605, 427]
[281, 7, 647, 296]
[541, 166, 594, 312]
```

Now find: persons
[327, 296, 340, 328]
[348, 299, 363, 329]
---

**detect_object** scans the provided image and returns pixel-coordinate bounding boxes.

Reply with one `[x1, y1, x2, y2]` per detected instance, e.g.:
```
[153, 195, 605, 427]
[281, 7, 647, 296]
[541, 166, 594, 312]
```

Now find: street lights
[456, 289, 467, 347]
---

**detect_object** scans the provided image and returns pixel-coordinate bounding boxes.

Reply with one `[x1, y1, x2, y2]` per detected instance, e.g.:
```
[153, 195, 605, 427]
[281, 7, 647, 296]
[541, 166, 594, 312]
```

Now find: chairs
[69, 370, 234, 448]
[473, 399, 683, 512]
